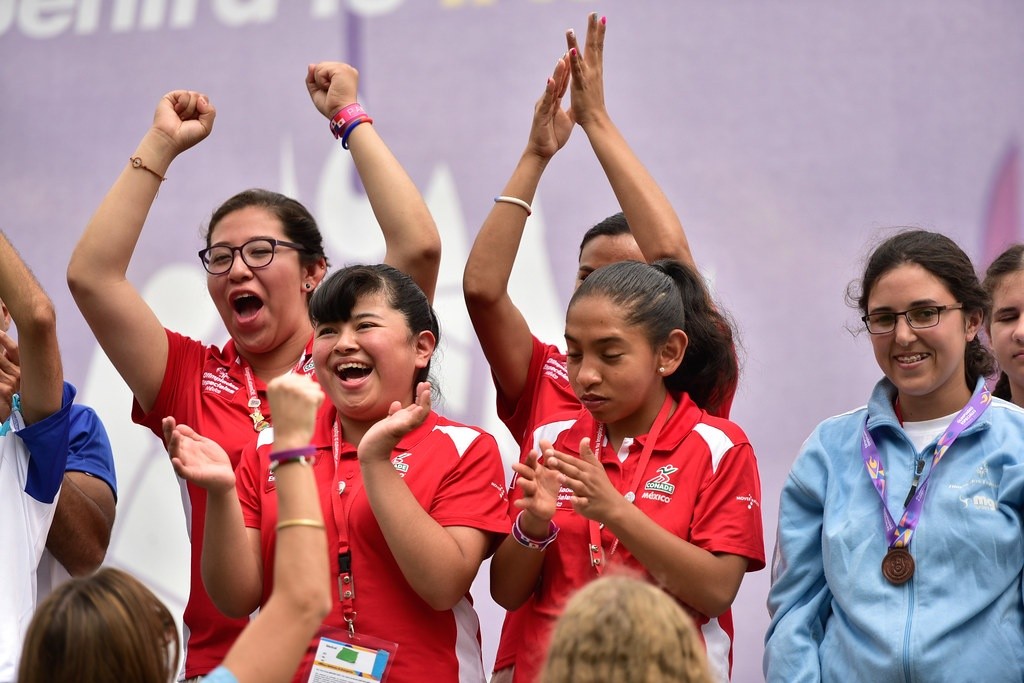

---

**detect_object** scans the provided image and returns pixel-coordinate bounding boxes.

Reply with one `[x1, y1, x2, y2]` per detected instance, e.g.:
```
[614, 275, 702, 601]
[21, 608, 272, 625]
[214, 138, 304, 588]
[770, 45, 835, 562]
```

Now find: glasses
[862, 303, 965, 335]
[198, 238, 305, 275]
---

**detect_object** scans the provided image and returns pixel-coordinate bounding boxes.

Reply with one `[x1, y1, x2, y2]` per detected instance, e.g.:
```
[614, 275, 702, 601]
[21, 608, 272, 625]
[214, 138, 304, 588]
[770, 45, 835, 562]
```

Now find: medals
[881, 550, 915, 585]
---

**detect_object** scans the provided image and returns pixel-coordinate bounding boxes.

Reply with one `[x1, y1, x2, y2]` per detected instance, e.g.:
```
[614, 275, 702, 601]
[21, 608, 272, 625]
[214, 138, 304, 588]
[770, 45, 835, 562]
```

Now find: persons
[0, 227, 117, 683]
[540, 574, 724, 683]
[980, 244, 1023, 408]
[69, 60, 442, 683]
[763, 230, 1023, 683]
[161, 262, 513, 683]
[462, 13, 738, 462]
[16, 368, 330, 683]
[489, 258, 767, 683]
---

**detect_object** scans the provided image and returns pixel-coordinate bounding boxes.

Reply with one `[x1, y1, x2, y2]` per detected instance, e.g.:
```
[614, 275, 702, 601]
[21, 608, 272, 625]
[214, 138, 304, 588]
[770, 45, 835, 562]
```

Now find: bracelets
[268, 445, 317, 471]
[512, 510, 560, 551]
[276, 519, 324, 530]
[341, 117, 373, 150]
[329, 102, 367, 139]
[494, 196, 531, 216]
[129, 157, 166, 182]
